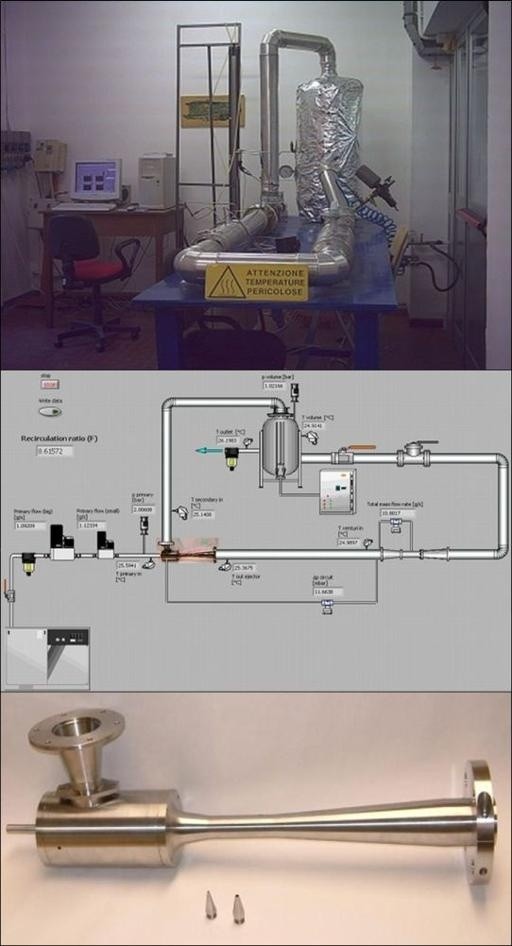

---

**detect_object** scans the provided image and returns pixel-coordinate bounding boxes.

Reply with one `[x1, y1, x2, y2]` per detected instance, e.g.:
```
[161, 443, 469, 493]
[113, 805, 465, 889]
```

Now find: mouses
[127, 205, 136, 211]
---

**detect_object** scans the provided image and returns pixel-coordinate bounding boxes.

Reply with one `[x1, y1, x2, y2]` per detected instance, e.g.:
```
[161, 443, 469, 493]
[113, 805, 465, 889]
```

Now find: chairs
[41, 214, 142, 352]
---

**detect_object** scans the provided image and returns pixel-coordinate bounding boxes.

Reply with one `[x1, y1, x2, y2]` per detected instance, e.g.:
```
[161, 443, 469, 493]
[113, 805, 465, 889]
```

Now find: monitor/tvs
[70, 158, 123, 201]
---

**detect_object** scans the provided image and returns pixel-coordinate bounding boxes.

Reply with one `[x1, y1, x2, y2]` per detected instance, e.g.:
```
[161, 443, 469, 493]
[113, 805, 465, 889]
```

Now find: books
[143, 150, 174, 157]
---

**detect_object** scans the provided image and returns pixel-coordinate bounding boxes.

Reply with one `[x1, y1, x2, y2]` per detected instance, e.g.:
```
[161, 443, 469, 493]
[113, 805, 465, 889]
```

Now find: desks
[128, 214, 399, 369]
[37, 207, 186, 329]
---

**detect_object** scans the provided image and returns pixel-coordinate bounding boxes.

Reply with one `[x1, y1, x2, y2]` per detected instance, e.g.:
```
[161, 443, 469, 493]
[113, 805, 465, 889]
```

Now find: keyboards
[51, 203, 117, 212]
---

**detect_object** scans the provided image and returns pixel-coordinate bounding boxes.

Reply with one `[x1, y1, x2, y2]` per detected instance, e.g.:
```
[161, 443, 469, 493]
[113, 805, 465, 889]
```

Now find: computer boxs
[138, 153, 176, 210]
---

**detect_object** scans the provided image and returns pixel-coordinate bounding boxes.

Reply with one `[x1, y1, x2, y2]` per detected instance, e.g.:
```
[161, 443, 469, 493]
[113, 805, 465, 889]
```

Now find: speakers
[121, 185, 131, 205]
